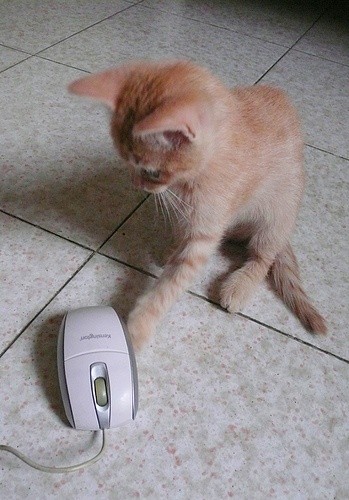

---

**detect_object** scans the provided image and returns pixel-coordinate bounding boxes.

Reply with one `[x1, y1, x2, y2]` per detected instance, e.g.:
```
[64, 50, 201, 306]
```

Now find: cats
[69, 60, 329, 348]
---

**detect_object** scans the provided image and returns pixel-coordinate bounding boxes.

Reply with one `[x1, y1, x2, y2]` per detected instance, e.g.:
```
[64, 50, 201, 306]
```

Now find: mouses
[55, 305, 140, 434]
